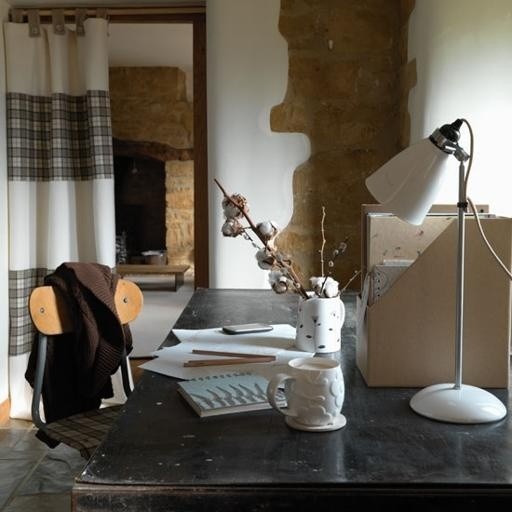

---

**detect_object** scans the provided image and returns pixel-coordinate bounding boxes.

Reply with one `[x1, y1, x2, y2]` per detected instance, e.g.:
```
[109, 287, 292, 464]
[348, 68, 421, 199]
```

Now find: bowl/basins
[142, 251, 167, 265]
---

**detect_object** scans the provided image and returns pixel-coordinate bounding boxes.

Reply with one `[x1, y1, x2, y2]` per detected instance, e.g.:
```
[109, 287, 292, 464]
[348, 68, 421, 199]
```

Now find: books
[137, 323, 316, 418]
[366, 213, 496, 305]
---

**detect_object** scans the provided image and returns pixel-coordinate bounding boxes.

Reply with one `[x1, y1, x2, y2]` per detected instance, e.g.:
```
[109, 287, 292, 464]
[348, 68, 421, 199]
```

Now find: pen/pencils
[184, 349, 276, 369]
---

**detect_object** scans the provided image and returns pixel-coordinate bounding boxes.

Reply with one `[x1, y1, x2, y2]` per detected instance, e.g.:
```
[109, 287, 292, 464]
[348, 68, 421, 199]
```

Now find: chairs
[28, 279, 144, 462]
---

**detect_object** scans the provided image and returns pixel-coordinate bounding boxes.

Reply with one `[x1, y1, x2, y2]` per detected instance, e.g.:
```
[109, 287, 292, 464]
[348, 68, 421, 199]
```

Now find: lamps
[363, 118, 507, 424]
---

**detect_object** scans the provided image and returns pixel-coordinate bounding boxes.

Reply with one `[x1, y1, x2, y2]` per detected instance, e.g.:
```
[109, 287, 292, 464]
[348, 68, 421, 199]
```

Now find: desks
[66, 285, 512, 509]
[117, 264, 191, 293]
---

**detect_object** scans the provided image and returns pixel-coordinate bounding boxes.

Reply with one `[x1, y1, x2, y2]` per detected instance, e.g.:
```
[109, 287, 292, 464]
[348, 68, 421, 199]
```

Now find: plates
[282, 410, 346, 433]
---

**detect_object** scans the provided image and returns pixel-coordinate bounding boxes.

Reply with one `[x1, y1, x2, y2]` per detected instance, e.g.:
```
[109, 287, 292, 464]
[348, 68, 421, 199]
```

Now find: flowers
[204, 175, 361, 302]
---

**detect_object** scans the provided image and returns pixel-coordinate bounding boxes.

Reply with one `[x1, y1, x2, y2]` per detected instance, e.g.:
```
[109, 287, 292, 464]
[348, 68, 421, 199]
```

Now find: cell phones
[222, 323, 273, 334]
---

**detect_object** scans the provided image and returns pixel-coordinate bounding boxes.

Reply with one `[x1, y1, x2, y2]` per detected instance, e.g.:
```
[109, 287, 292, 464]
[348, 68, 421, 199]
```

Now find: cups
[267, 356, 346, 426]
